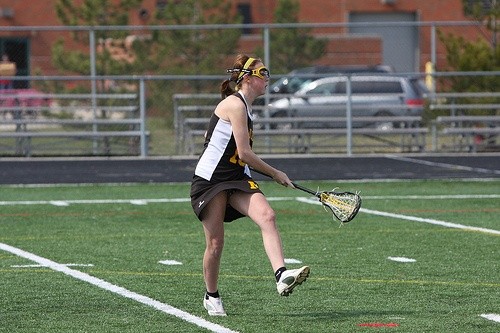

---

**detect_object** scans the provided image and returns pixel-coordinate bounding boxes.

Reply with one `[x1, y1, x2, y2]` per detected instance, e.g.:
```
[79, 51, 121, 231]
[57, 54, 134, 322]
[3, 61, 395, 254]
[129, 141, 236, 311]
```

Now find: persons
[190, 54, 310, 316]
[0, 55, 16, 88]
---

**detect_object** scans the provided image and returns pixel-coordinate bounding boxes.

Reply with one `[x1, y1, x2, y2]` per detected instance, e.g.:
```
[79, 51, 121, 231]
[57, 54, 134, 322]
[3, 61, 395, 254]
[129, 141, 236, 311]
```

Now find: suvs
[264, 75, 431, 133]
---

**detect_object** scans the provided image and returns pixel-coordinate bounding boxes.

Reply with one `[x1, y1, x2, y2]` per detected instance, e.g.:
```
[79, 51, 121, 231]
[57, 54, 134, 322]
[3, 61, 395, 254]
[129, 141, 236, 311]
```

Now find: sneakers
[276, 266, 310, 297]
[203, 292, 228, 316]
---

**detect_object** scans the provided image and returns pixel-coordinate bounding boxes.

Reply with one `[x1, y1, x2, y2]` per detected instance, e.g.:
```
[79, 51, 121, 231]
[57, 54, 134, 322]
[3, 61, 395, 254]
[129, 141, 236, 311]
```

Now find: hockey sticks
[249, 168, 362, 228]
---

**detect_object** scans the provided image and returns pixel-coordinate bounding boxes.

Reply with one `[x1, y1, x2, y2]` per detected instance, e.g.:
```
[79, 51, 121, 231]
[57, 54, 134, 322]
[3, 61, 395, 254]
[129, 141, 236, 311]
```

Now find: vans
[268, 65, 398, 97]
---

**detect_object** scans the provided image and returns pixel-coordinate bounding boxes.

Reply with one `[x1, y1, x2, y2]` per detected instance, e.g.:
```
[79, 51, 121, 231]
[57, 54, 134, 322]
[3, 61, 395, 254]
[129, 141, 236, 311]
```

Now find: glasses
[226, 67, 270, 80]
